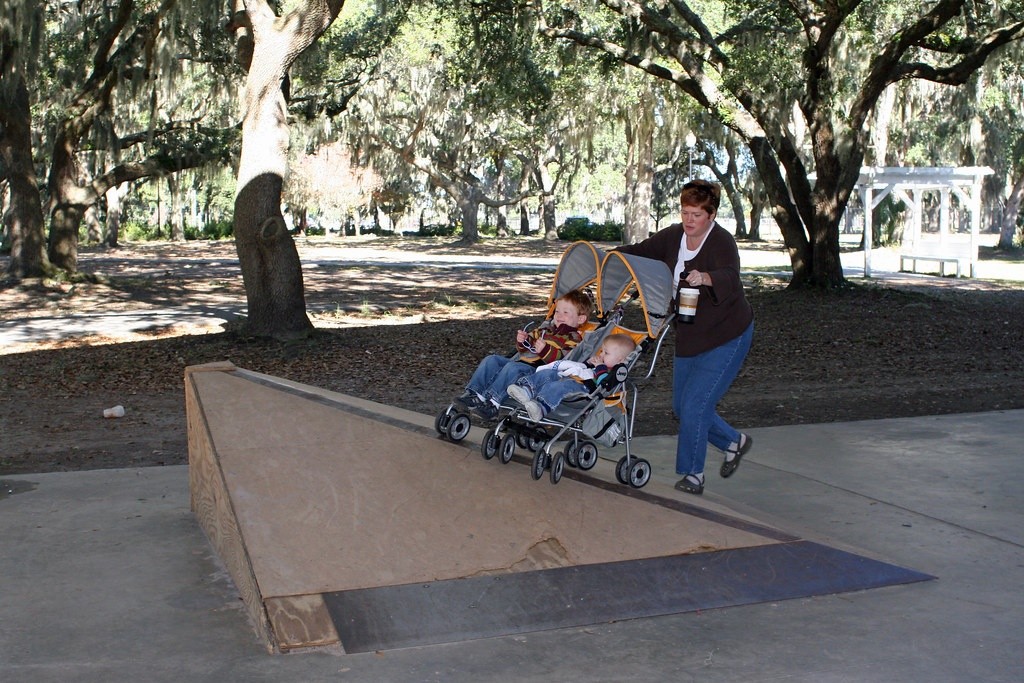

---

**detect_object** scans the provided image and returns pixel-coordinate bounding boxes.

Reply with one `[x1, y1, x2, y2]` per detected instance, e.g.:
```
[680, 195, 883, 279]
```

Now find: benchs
[897, 234, 973, 278]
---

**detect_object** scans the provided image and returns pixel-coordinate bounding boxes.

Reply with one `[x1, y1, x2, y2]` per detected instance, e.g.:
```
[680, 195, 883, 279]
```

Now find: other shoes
[524, 400, 543, 422]
[507, 384, 531, 406]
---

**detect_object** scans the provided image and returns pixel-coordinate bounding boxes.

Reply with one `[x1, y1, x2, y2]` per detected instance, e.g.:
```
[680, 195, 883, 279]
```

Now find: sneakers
[453, 396, 482, 412]
[471, 400, 500, 421]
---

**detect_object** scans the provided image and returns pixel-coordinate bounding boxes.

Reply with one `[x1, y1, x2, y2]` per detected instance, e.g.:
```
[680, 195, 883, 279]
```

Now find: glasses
[520, 321, 545, 355]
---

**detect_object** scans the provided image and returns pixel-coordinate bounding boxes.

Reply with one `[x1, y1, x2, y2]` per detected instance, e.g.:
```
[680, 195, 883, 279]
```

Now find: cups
[676, 288, 700, 326]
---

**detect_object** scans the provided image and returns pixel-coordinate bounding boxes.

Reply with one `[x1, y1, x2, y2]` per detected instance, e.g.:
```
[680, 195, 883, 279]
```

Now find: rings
[692, 281, 696, 284]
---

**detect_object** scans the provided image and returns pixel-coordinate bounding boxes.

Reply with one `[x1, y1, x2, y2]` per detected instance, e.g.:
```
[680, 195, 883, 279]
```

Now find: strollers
[436, 241, 695, 489]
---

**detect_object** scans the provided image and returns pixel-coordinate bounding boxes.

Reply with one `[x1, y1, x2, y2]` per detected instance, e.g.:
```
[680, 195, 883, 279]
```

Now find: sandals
[719, 431, 753, 478]
[674, 473, 705, 495]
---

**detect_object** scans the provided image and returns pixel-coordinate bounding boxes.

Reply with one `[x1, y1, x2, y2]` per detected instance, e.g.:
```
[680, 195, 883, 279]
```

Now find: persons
[453, 290, 591, 420]
[507, 334, 637, 422]
[604, 179, 753, 495]
